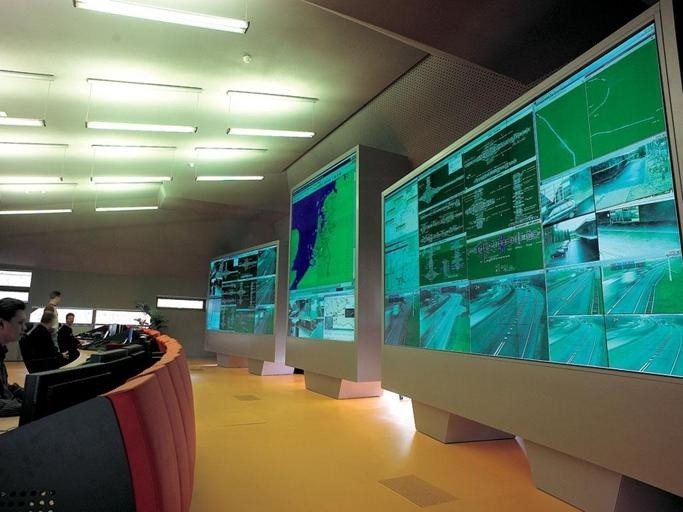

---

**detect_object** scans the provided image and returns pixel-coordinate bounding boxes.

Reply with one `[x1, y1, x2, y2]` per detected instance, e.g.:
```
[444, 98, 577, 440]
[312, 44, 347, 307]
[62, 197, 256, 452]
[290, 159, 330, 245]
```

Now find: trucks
[392, 305, 402, 318]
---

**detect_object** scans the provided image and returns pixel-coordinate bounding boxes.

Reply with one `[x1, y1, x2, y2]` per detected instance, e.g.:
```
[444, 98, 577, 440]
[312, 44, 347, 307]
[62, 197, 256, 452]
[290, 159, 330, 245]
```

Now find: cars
[620, 271, 637, 285]
[551, 239, 570, 260]
[540, 198, 577, 226]
[521, 285, 532, 292]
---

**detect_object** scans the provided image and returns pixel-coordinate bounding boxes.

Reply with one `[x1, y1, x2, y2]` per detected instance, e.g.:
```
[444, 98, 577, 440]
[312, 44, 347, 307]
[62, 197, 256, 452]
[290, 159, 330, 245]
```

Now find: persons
[29, 290, 62, 323]
[21, 310, 72, 372]
[0, 297, 31, 417]
[34, 306, 60, 352]
[57, 312, 82, 361]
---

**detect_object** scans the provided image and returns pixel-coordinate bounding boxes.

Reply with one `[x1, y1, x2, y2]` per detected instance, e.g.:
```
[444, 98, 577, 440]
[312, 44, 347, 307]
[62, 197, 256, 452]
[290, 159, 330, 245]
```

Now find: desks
[0, 346, 98, 437]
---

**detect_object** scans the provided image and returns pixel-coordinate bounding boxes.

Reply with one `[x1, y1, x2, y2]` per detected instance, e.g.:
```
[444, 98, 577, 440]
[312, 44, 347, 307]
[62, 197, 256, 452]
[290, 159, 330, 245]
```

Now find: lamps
[3, 142, 70, 183]
[1, 180, 79, 215]
[225, 89, 319, 140]
[72, 0, 250, 35]
[93, 181, 165, 213]
[90, 144, 179, 183]
[83, 77, 203, 133]
[0, 69, 56, 128]
[194, 146, 269, 181]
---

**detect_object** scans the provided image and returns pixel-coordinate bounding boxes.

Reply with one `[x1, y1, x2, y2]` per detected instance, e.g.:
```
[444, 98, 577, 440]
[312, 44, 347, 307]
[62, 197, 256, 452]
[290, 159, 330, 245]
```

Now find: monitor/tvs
[17, 324, 164, 428]
